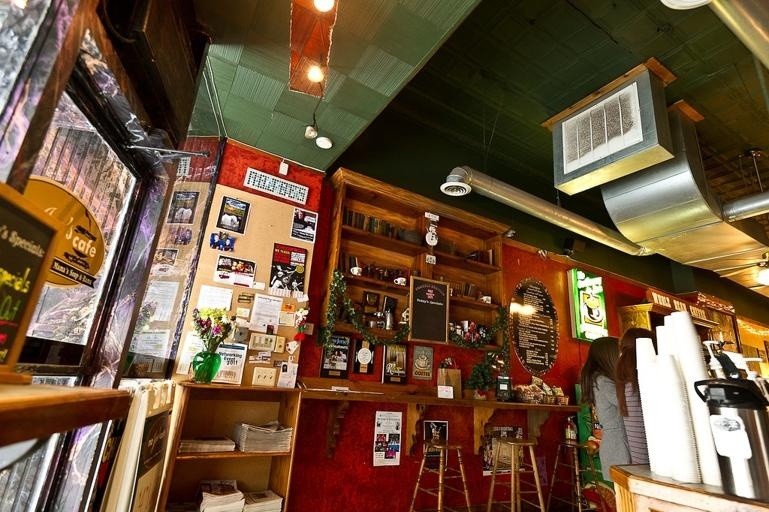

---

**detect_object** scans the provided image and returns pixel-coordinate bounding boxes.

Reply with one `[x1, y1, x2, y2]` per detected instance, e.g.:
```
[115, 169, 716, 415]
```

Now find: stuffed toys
[292, 307, 308, 342]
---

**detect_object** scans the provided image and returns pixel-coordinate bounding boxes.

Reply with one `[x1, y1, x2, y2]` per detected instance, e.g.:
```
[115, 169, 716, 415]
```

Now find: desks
[609, 464, 769, 512]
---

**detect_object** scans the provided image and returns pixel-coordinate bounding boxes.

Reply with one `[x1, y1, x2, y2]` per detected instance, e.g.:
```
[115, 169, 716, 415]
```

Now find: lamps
[301, 0, 335, 141]
[755, 251, 769, 286]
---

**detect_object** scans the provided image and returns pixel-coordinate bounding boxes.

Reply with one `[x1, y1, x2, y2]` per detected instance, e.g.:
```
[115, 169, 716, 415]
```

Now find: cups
[417, 355, 432, 369]
[585, 295, 604, 324]
[393, 277, 407, 286]
[480, 295, 492, 304]
[635, 311, 724, 489]
[350, 266, 365, 277]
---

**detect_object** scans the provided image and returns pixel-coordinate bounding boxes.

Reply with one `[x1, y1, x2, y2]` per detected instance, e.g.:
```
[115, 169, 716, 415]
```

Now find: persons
[615, 327, 656, 465]
[211, 235, 232, 251]
[579, 336, 630, 483]
[220, 208, 240, 230]
[272, 265, 302, 291]
[327, 347, 346, 367]
[172, 199, 192, 223]
[170, 228, 192, 246]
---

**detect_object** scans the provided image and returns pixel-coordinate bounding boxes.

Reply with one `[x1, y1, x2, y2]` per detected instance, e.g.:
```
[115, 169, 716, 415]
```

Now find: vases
[192, 352, 221, 384]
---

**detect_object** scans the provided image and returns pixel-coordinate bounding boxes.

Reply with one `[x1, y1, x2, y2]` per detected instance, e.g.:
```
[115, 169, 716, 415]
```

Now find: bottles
[376, 310, 395, 331]
[693, 376, 769, 503]
[367, 263, 384, 280]
[448, 322, 463, 338]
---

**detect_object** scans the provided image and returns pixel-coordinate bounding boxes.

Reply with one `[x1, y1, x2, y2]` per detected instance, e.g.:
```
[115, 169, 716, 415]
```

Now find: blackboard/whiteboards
[508, 277, 561, 376]
[0, 180, 65, 385]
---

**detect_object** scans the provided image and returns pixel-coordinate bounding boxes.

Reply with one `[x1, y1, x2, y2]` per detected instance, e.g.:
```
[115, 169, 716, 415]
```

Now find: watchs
[356, 340, 372, 375]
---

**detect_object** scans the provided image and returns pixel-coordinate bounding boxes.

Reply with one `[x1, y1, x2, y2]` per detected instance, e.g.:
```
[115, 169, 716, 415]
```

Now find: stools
[408, 441, 472, 512]
[486, 435, 545, 512]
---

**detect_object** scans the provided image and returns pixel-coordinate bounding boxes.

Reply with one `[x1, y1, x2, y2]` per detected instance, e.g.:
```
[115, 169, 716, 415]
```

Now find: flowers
[190, 307, 238, 383]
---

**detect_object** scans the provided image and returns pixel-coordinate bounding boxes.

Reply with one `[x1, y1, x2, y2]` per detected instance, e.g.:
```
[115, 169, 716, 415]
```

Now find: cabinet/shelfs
[157, 382, 302, 512]
[322, 167, 512, 354]
